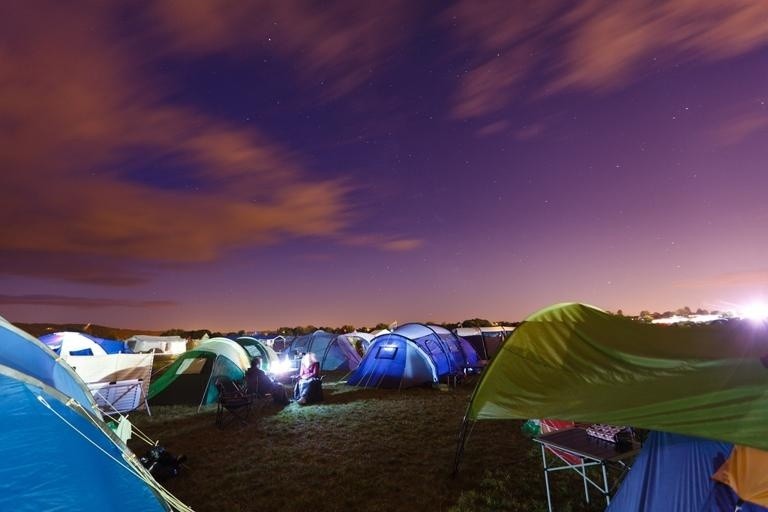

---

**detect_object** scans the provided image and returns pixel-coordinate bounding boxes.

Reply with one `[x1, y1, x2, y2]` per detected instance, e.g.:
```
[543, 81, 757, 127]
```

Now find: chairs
[214, 375, 274, 431]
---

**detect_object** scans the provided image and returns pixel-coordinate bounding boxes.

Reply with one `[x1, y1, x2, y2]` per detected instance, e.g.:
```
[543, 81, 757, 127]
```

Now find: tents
[36, 322, 519, 414]
[0, 316, 193, 512]
[450, 301, 767, 511]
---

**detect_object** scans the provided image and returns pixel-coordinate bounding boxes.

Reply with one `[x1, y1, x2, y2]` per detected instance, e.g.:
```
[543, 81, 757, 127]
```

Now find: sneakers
[296, 396, 308, 404]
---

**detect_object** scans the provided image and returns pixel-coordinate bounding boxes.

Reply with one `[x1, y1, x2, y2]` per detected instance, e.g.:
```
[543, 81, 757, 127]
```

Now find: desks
[531, 426, 640, 512]
